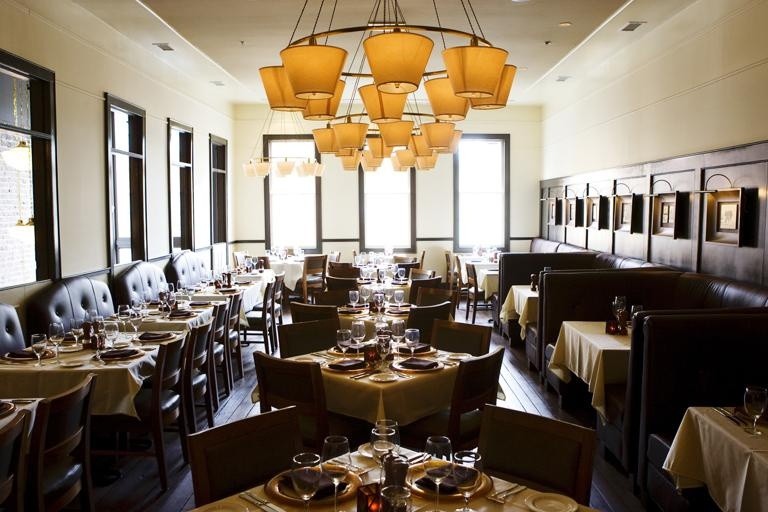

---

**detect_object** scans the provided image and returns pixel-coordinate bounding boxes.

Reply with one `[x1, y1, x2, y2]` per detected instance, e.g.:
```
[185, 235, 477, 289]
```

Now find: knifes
[712, 406, 747, 428]
[240, 488, 286, 511]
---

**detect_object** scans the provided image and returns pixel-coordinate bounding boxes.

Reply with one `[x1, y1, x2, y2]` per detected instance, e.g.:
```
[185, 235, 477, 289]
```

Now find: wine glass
[743, 386, 767, 437]
[30, 249, 643, 373]
[294, 419, 493, 511]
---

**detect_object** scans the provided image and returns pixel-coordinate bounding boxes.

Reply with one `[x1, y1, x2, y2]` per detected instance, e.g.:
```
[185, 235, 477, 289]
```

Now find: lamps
[0, 78, 35, 227]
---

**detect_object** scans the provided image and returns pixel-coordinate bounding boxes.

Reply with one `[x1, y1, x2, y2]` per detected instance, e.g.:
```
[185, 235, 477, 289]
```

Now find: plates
[0, 401, 17, 419]
[4, 350, 56, 360]
[265, 463, 365, 505]
[367, 373, 400, 383]
[525, 492, 578, 511]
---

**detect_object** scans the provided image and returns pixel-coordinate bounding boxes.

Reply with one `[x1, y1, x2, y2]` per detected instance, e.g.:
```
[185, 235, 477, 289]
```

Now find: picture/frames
[546, 187, 744, 248]
